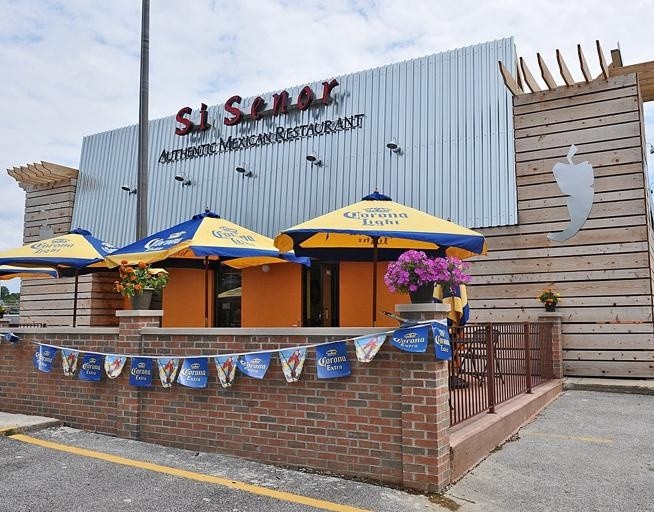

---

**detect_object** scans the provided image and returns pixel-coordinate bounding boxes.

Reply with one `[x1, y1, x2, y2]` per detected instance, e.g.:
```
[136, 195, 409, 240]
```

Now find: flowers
[538, 288, 561, 305]
[110, 259, 169, 298]
[384, 249, 472, 295]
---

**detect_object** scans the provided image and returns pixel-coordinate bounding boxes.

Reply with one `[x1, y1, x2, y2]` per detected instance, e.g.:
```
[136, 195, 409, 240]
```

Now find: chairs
[458, 329, 505, 386]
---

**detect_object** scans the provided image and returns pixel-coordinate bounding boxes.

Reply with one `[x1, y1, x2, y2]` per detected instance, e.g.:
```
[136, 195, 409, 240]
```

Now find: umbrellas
[274, 187, 489, 327]
[106, 206, 312, 327]
[0, 224, 119, 326]
[433, 217, 470, 388]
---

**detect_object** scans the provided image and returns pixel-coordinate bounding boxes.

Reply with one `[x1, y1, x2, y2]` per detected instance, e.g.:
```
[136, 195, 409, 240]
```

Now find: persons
[287, 349, 301, 380]
[364, 335, 379, 360]
[111, 357, 124, 374]
[164, 360, 177, 383]
[66, 351, 75, 375]
[223, 356, 234, 383]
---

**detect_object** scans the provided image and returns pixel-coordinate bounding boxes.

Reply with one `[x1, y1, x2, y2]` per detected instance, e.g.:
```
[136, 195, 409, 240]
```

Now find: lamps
[174, 173, 190, 186]
[386, 139, 402, 154]
[305, 150, 322, 167]
[237, 163, 251, 176]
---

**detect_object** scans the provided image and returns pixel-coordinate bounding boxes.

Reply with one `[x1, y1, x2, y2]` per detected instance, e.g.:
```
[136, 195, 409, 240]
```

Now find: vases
[546, 304, 556, 312]
[409, 282, 434, 303]
[130, 287, 154, 311]
